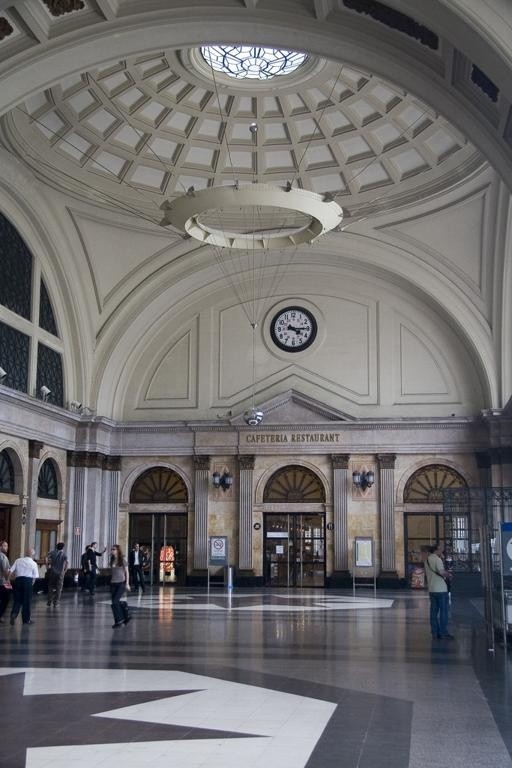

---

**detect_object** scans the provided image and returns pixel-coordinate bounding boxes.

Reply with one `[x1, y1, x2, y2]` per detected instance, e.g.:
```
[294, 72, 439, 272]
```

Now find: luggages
[110, 600, 130, 626]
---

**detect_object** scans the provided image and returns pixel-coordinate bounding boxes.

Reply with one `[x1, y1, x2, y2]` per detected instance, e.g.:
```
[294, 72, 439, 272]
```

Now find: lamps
[40, 385, 52, 403]
[212, 470, 234, 493]
[71, 398, 83, 413]
[351, 466, 375, 493]
[1, 365, 8, 383]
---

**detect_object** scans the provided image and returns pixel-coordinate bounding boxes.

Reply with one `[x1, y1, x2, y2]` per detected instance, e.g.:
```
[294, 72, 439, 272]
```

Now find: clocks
[269, 305, 319, 353]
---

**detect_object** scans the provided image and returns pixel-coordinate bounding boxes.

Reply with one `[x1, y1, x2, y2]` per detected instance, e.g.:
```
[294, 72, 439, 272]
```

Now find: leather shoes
[22, 618, 33, 624]
[112, 620, 126, 629]
[8, 615, 16, 626]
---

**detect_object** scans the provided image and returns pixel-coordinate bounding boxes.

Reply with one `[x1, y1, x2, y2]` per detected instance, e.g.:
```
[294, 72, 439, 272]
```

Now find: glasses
[111, 548, 117, 551]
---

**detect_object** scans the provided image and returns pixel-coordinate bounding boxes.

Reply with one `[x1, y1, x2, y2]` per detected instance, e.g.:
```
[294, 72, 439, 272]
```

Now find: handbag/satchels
[444, 578, 453, 593]
[44, 567, 52, 584]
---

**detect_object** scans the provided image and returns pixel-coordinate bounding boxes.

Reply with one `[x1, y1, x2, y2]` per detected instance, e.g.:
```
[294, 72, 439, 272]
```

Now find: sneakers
[46, 601, 51, 607]
[53, 603, 60, 608]
[439, 632, 454, 641]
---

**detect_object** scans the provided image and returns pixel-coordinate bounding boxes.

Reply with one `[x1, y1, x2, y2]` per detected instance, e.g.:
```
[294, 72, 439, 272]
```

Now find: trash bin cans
[224, 565, 234, 587]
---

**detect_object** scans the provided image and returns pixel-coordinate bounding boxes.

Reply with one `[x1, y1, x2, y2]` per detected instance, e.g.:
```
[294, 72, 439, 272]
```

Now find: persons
[425, 543, 454, 640]
[108, 545, 130, 629]
[9, 548, 39, 626]
[80, 545, 92, 592]
[80, 542, 107, 595]
[130, 543, 146, 592]
[45, 542, 69, 607]
[0, 539, 10, 622]
